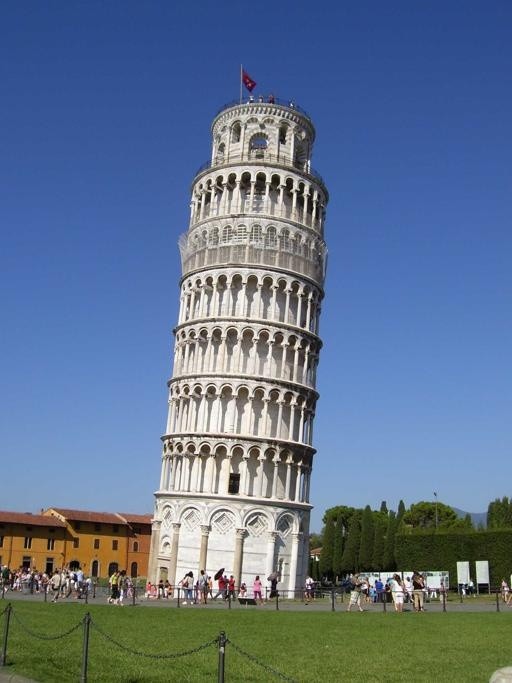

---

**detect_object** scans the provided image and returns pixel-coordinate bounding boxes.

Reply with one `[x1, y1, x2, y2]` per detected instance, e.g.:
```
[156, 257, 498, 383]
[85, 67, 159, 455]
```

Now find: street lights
[431, 491, 438, 530]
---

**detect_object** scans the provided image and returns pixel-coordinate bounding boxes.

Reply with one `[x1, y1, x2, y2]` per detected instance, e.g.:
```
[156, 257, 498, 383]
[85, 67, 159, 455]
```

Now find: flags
[243, 70, 256, 92]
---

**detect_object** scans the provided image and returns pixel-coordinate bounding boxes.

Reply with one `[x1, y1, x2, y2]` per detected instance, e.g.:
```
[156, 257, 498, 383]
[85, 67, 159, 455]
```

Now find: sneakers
[4, 588, 89, 603]
[182, 602, 187, 605]
[395, 610, 398, 612]
[190, 601, 194, 603]
[420, 609, 425, 612]
[400, 610, 402, 612]
[346, 610, 351, 612]
[360, 610, 367, 611]
[416, 609, 419, 612]
[108, 597, 124, 606]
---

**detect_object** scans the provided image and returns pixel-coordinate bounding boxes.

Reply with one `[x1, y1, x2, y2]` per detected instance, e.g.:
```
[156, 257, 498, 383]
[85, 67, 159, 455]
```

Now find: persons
[461, 578, 476, 599]
[1, 565, 93, 602]
[248, 91, 274, 104]
[253, 576, 266, 606]
[146, 579, 171, 600]
[178, 570, 247, 605]
[305, 575, 314, 602]
[107, 569, 135, 607]
[499, 576, 511, 608]
[268, 579, 280, 602]
[430, 582, 448, 603]
[323, 570, 426, 612]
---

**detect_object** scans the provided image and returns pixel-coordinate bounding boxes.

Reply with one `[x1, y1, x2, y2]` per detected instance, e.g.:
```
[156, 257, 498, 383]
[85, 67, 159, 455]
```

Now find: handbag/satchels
[184, 581, 189, 586]
[348, 582, 354, 590]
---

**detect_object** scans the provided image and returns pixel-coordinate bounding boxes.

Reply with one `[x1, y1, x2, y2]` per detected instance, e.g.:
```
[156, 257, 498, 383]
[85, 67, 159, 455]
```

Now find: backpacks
[203, 575, 209, 588]
